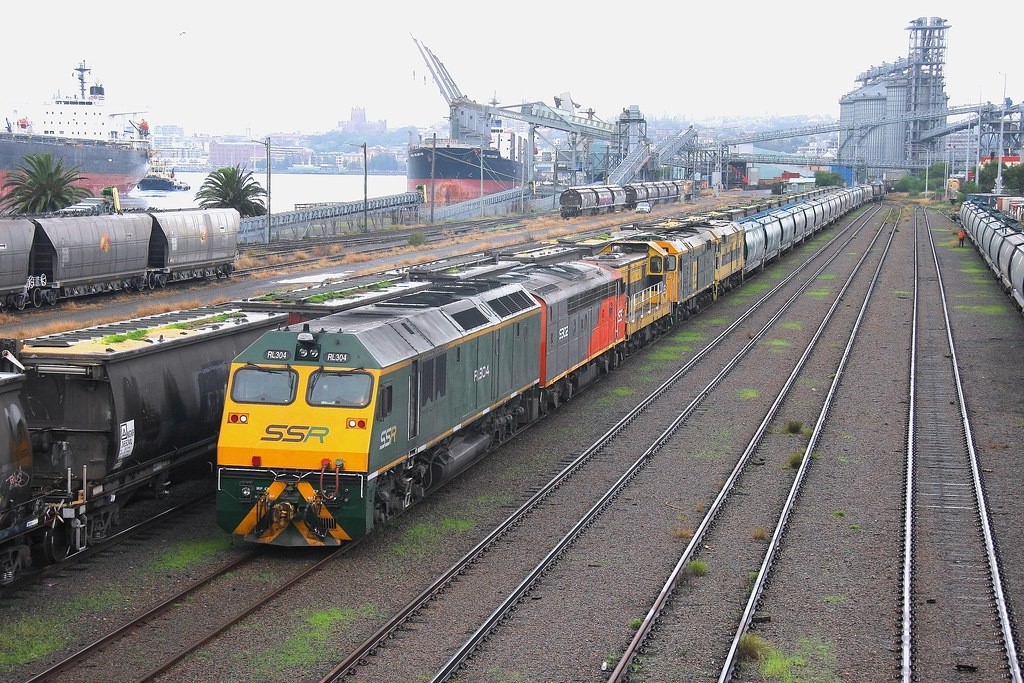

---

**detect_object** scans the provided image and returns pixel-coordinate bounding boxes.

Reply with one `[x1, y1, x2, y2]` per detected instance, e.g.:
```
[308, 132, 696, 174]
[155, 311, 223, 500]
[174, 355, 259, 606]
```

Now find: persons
[958, 230, 965, 247]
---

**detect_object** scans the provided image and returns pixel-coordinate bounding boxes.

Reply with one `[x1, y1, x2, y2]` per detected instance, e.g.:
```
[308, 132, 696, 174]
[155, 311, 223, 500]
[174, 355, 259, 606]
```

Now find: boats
[137, 174, 190, 191]
[0, 60, 157, 211]
[408, 32, 538, 208]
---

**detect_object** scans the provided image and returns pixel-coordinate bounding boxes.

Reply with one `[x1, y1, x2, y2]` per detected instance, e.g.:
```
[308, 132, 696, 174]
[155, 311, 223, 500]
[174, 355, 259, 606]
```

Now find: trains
[0, 178, 894, 589]
[960, 196, 1024, 318]
[0, 208, 240, 311]
[558, 180, 696, 220]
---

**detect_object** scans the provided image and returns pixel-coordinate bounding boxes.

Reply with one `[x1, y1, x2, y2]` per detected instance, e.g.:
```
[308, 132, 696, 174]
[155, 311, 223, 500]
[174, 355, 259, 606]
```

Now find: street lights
[350, 142, 367, 233]
[923, 146, 928, 199]
[252, 137, 271, 244]
[991, 71, 1007, 194]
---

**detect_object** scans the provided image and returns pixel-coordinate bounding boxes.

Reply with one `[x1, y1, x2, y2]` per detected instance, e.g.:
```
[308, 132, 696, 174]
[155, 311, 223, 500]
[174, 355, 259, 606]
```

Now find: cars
[636, 202, 651, 213]
